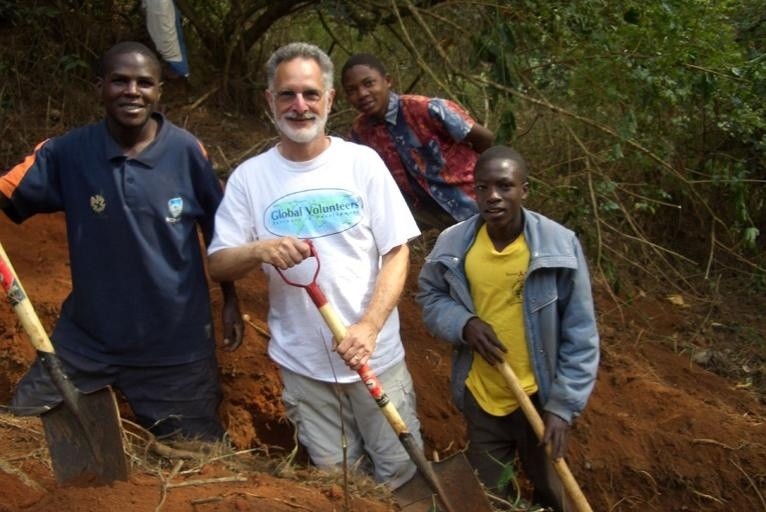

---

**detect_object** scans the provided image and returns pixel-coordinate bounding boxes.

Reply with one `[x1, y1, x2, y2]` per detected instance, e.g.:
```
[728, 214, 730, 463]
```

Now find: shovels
[1, 239, 129, 489]
[273, 239, 495, 511]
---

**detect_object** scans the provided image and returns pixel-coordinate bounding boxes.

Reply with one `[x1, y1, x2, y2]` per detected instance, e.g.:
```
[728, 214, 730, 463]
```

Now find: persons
[1, 40, 244, 447]
[342, 53, 499, 232]
[203, 44, 427, 493]
[415, 147, 605, 510]
